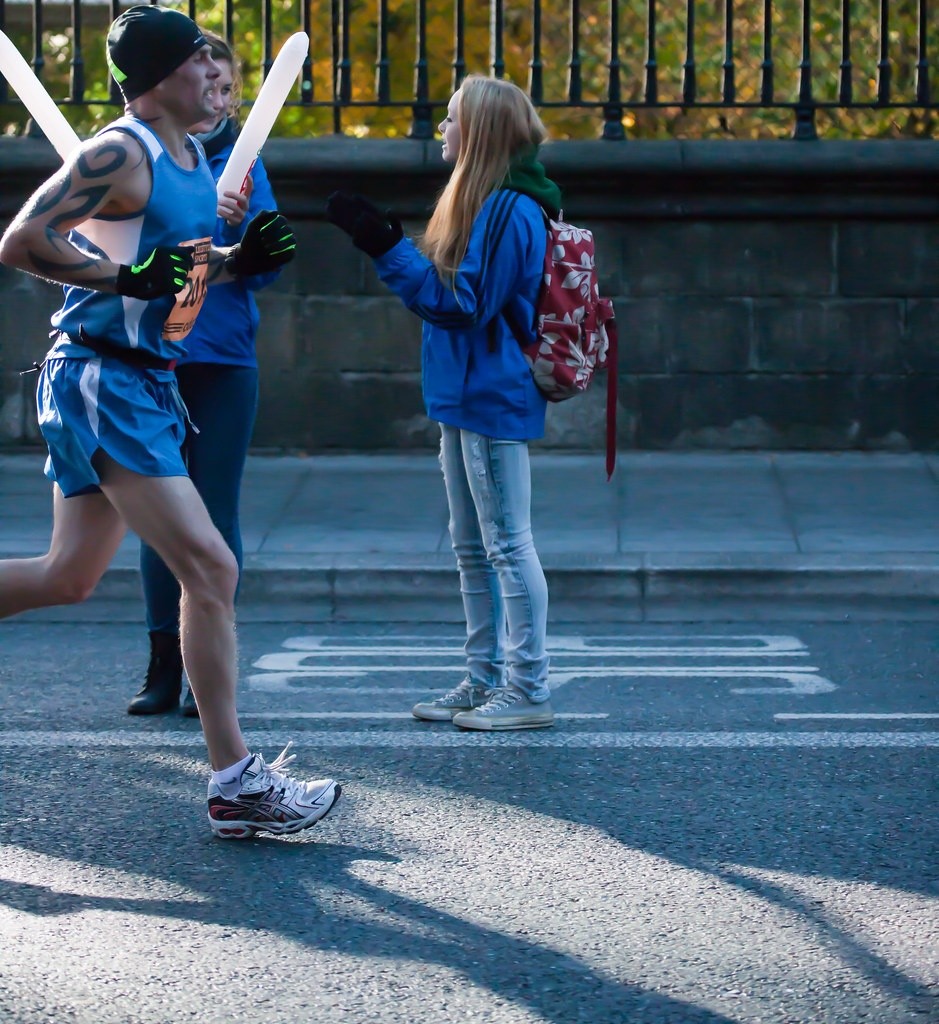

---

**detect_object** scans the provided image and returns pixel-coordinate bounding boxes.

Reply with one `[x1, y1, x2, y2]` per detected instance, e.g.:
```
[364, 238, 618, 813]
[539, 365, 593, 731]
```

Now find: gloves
[224, 209, 296, 278]
[324, 191, 403, 259]
[116, 245, 195, 301]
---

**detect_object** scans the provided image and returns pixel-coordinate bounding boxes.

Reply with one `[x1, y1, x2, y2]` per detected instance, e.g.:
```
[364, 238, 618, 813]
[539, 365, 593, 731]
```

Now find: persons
[323, 75, 561, 731]
[0, 5, 343, 839]
[127, 31, 285, 717]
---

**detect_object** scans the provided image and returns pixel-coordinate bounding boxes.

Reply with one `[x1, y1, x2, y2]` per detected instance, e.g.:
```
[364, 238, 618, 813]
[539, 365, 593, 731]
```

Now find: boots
[127, 630, 183, 714]
[183, 687, 199, 717]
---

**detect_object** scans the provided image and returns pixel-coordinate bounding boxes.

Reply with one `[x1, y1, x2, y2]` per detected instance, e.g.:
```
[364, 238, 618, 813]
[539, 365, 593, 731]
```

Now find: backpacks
[486, 209, 618, 481]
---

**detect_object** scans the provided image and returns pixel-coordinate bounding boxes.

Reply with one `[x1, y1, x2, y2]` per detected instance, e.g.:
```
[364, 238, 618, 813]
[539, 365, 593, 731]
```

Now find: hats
[107, 5, 209, 103]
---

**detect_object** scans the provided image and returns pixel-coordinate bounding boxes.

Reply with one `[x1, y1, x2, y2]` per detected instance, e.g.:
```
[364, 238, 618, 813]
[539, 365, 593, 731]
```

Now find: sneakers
[453, 683, 555, 730]
[413, 676, 507, 720]
[207, 741, 342, 839]
